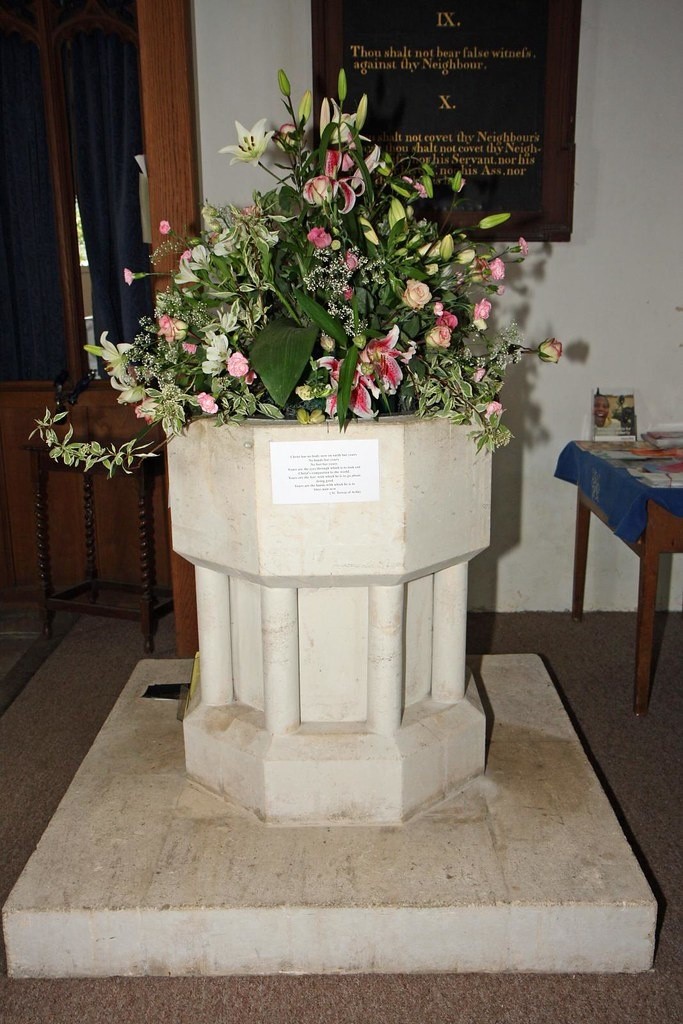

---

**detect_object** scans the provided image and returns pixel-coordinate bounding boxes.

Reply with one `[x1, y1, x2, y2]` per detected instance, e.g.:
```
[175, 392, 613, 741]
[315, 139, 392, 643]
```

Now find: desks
[18, 438, 174, 656]
[553, 440, 683, 718]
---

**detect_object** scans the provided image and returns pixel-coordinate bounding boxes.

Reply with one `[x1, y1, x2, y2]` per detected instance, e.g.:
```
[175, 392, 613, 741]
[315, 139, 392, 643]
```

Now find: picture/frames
[311, 0, 582, 243]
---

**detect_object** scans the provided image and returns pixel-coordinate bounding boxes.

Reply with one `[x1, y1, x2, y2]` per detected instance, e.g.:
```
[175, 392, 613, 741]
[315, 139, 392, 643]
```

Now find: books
[576, 387, 683, 489]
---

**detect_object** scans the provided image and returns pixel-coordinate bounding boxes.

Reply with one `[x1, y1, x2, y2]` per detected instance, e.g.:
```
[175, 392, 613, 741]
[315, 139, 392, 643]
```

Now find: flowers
[26, 67, 563, 480]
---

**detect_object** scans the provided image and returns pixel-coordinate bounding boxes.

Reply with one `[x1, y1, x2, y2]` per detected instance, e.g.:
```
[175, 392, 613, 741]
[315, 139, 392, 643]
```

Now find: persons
[595, 396, 622, 436]
